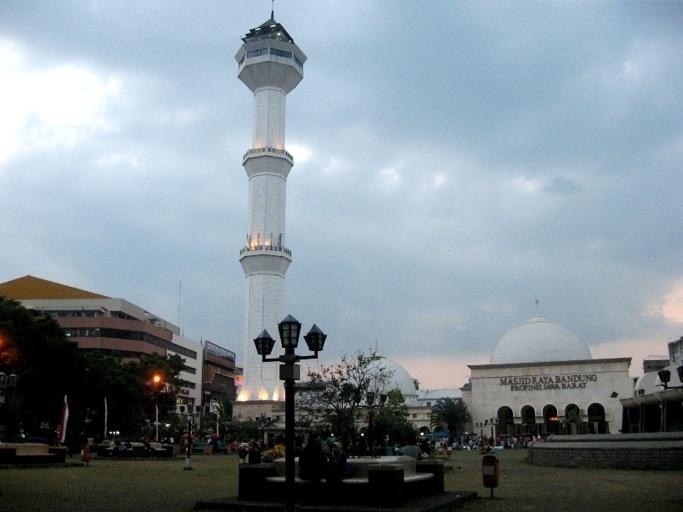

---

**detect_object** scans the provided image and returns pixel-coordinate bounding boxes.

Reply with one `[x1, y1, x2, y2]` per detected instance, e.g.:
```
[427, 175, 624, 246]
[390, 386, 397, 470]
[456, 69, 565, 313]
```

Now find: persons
[79, 432, 286, 468]
[421, 434, 541, 460]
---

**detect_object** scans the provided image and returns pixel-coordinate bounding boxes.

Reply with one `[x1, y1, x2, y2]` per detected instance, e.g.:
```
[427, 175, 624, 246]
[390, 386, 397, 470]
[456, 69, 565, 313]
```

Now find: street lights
[253, 311, 329, 504]
[180, 404, 202, 472]
